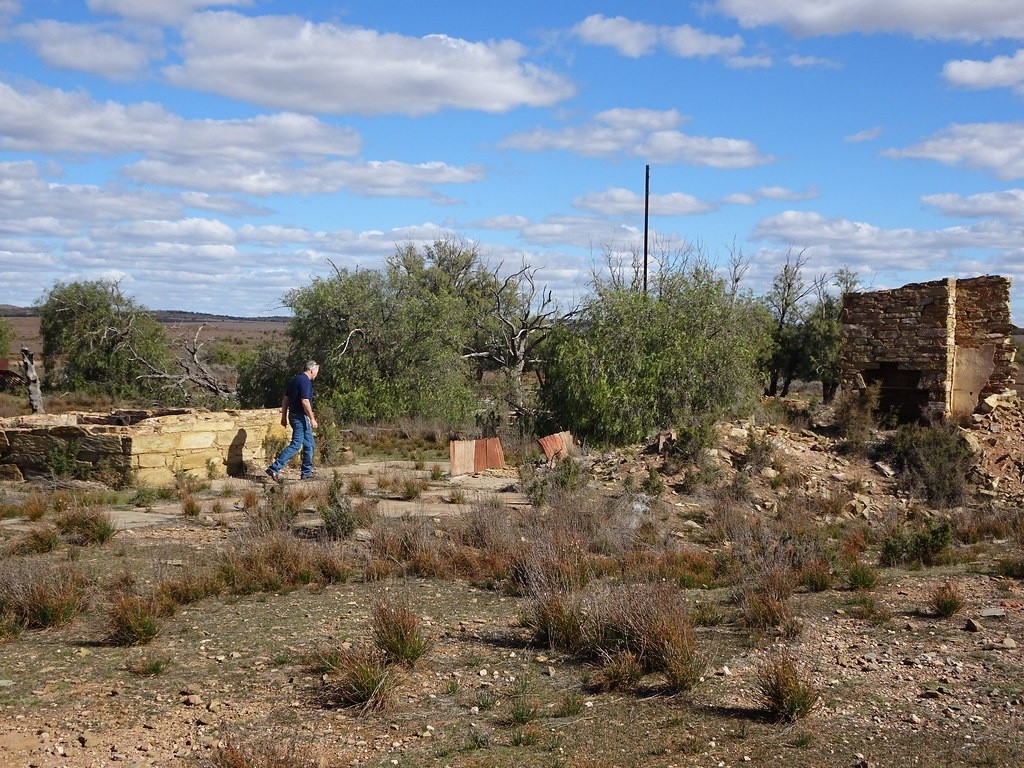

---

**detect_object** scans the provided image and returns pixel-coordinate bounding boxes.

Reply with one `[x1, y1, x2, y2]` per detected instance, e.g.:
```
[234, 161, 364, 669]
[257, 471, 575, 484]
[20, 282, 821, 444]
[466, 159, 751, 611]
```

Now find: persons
[266, 359, 322, 481]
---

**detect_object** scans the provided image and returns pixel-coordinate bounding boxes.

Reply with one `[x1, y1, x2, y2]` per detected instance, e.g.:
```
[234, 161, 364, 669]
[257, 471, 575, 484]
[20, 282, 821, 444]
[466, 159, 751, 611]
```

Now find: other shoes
[265, 468, 279, 481]
[301, 473, 320, 480]
